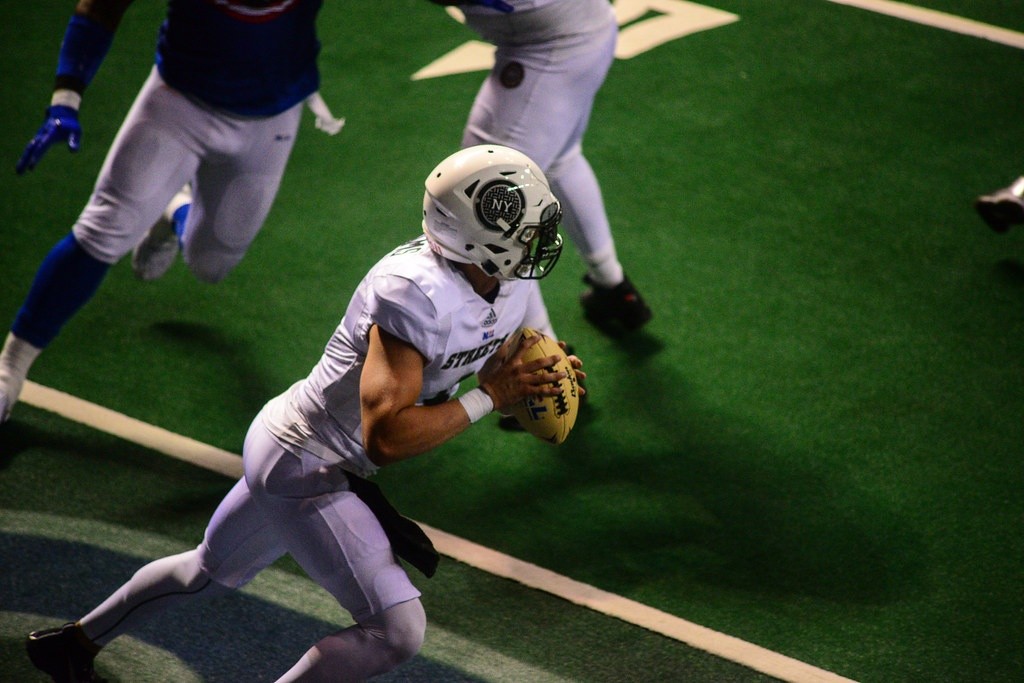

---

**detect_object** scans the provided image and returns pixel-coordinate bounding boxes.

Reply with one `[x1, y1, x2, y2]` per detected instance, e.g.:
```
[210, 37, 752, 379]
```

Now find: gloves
[14, 105, 83, 178]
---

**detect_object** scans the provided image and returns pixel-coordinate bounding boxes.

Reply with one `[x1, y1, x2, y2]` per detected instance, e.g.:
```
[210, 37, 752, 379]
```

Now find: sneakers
[131, 182, 192, 282]
[27, 619, 113, 683]
[0, 370, 24, 420]
[582, 271, 653, 336]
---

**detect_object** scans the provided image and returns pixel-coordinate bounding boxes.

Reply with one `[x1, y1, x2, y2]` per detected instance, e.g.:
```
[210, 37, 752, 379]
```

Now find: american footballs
[504, 326, 581, 446]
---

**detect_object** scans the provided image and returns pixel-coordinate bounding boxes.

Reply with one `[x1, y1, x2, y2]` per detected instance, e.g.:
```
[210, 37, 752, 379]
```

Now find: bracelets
[457, 386, 494, 424]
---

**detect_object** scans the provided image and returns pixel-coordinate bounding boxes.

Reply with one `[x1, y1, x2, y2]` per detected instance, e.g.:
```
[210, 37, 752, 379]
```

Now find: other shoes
[974, 172, 1024, 235]
[498, 343, 587, 432]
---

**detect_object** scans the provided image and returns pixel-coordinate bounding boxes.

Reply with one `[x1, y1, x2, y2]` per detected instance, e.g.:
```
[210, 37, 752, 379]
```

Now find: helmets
[421, 144, 563, 280]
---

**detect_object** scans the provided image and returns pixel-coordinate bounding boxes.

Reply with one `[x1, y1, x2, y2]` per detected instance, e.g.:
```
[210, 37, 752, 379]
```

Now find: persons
[0, 0, 322, 423]
[976, 173, 1024, 233]
[26, 143, 586, 683]
[426, 0, 654, 325]
